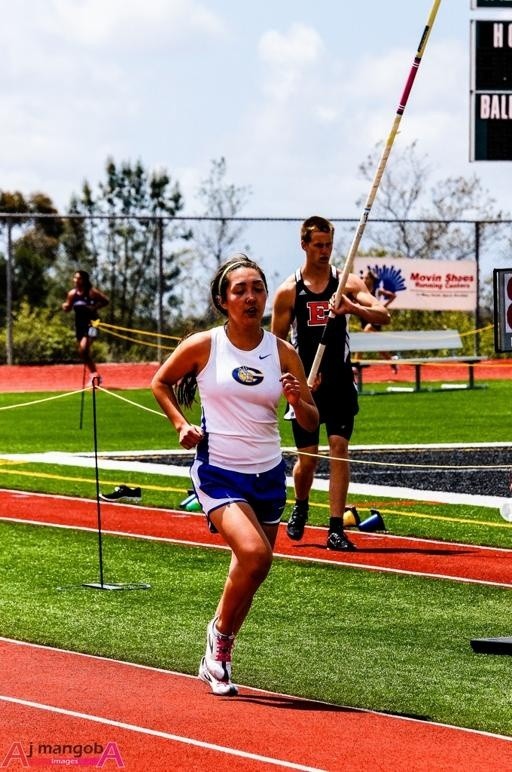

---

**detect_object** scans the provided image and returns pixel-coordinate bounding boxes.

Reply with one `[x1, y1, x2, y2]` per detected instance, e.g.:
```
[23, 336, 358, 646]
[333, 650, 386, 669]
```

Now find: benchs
[343, 328, 489, 396]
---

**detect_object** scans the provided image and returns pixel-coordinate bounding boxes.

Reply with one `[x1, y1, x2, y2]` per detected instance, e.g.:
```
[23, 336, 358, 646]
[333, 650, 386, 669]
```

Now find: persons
[61, 270, 110, 387]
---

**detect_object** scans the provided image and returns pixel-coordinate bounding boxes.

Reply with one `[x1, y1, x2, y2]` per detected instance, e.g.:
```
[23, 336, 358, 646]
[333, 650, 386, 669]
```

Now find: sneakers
[197, 616, 238, 696]
[86, 372, 102, 387]
[98, 485, 142, 503]
[287, 505, 308, 541]
[327, 531, 355, 552]
